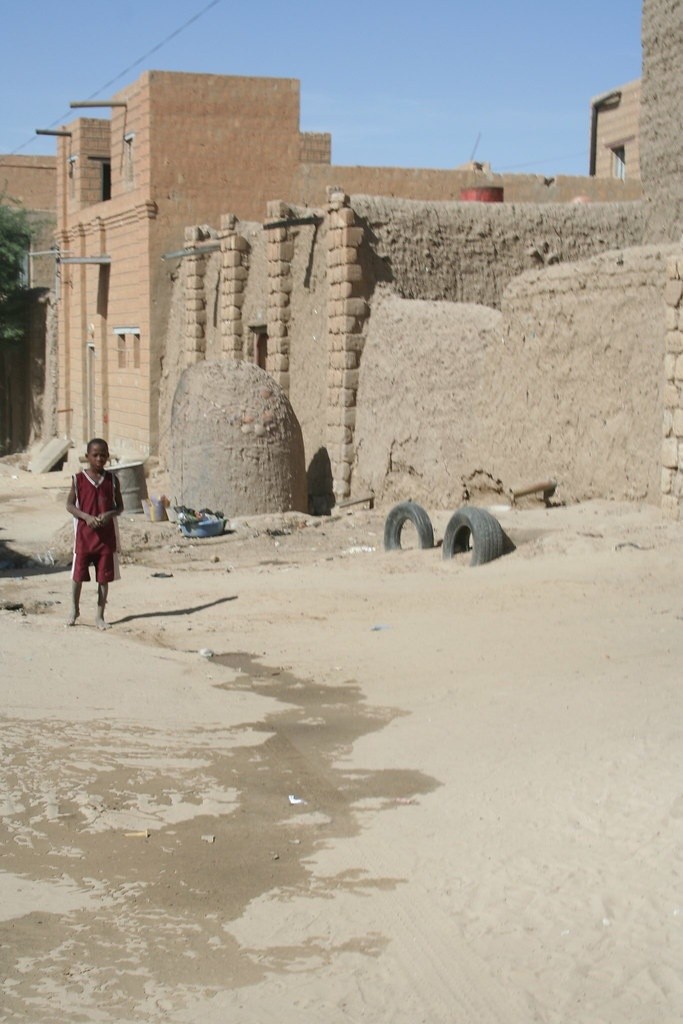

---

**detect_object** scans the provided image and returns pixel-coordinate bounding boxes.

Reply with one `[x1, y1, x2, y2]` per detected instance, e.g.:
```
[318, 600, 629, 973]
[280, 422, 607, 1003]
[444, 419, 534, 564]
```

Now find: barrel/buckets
[113, 466, 148, 513]
[141, 499, 167, 521]
[461, 187, 503, 203]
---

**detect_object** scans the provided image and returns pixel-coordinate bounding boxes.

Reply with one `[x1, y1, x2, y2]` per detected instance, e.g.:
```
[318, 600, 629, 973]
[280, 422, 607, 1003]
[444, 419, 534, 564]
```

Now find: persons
[63, 439, 124, 631]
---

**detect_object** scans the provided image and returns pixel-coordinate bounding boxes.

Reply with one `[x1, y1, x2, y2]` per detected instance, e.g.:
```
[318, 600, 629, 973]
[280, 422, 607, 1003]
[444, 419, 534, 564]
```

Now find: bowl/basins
[181, 519, 227, 538]
